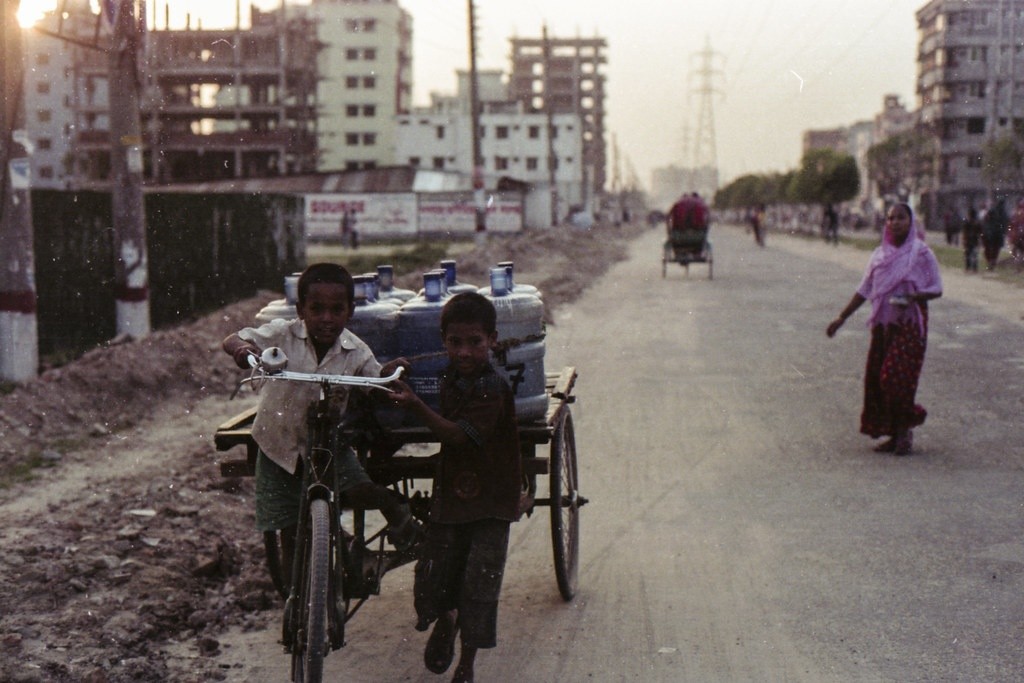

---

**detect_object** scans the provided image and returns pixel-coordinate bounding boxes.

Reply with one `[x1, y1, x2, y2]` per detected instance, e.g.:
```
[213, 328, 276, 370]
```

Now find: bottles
[376, 265, 416, 300]
[417, 260, 478, 295]
[478, 261, 542, 299]
[255, 272, 304, 329]
[397, 269, 458, 425]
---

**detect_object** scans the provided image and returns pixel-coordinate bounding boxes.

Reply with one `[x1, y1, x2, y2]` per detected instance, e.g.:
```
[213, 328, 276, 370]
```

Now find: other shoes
[873, 442, 898, 452]
[893, 431, 913, 456]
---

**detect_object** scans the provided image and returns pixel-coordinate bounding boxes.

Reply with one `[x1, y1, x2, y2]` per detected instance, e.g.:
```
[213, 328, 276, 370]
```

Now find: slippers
[425, 611, 459, 674]
[389, 502, 417, 552]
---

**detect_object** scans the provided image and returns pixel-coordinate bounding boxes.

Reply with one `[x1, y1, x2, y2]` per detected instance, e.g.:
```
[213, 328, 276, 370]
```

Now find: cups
[468, 268, 547, 427]
[352, 272, 404, 429]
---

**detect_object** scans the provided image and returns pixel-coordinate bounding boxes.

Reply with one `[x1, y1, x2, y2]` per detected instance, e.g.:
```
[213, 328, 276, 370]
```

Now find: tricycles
[213, 345, 590, 683]
[660, 227, 714, 281]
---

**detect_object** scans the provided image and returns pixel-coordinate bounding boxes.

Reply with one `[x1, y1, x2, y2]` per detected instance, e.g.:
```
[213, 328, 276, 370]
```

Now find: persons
[945, 198, 1024, 273]
[341, 208, 360, 248]
[826, 201, 942, 456]
[667, 192, 710, 261]
[222, 263, 411, 591]
[389, 291, 521, 683]
